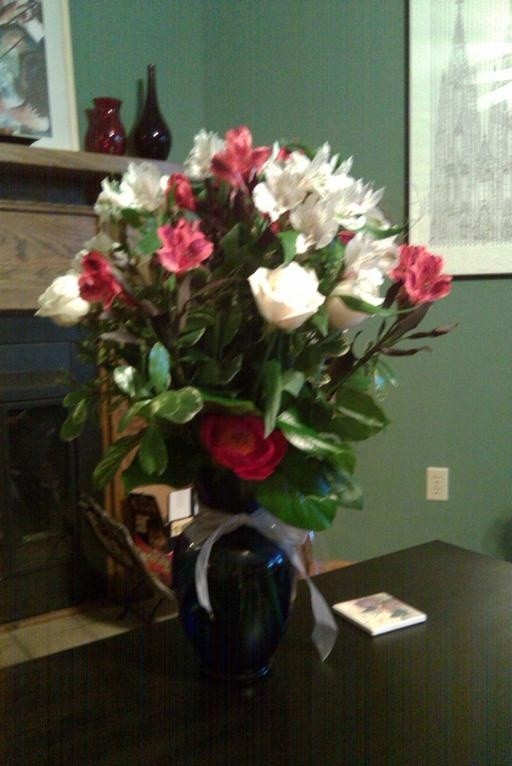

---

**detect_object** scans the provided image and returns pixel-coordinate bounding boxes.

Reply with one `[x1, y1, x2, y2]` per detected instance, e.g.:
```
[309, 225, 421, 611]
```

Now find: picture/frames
[403, 0, 512, 280]
[0, 0, 80, 152]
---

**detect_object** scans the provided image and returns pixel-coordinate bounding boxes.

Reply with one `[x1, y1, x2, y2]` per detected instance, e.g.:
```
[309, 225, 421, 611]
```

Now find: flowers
[32, 124, 461, 533]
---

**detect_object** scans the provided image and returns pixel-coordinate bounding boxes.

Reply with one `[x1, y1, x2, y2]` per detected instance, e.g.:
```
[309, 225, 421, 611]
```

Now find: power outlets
[426, 467, 449, 501]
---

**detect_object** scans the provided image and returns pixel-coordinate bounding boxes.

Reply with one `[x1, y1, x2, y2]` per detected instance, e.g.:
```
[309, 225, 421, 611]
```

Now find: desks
[2, 539, 512, 762]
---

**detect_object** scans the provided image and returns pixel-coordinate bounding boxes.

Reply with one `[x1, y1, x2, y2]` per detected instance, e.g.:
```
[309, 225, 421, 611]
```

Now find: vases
[133, 62, 170, 161]
[86, 96, 126, 155]
[171, 469, 293, 686]
